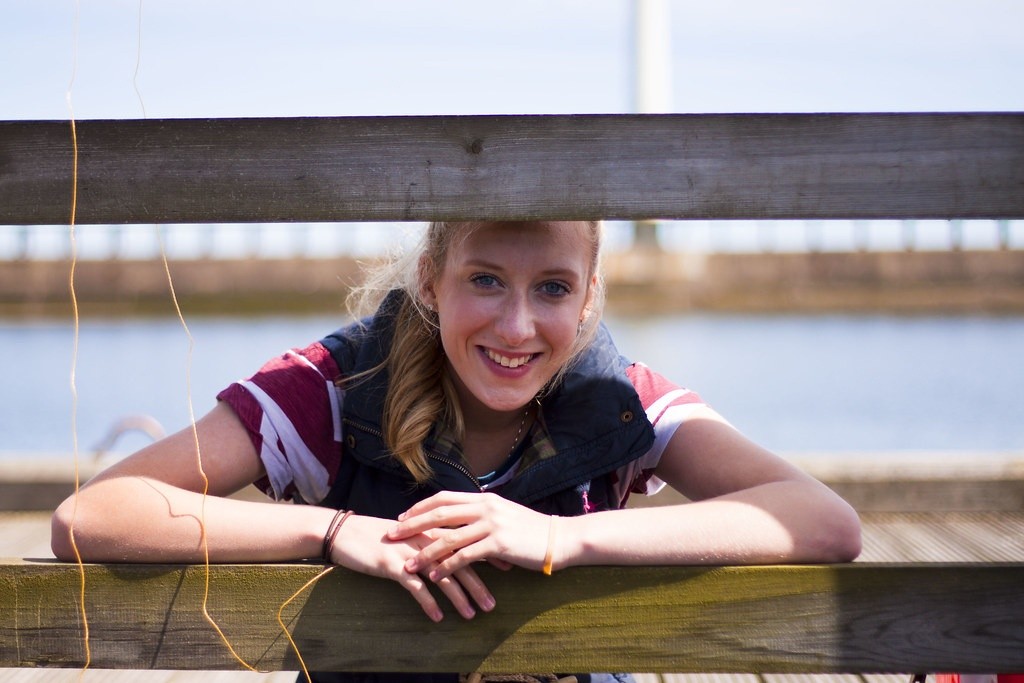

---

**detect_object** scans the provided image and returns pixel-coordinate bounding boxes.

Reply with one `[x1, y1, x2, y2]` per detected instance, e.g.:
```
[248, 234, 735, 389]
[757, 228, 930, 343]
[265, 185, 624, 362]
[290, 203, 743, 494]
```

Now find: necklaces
[510, 396, 533, 455]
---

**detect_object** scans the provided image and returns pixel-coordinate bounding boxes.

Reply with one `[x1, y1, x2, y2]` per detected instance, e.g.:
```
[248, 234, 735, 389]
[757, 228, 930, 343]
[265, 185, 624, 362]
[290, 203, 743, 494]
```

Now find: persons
[49, 222, 864, 683]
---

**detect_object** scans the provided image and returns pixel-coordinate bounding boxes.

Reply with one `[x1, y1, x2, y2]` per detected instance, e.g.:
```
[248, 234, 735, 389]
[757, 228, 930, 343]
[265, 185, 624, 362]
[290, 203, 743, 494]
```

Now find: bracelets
[320, 506, 357, 562]
[542, 513, 561, 577]
